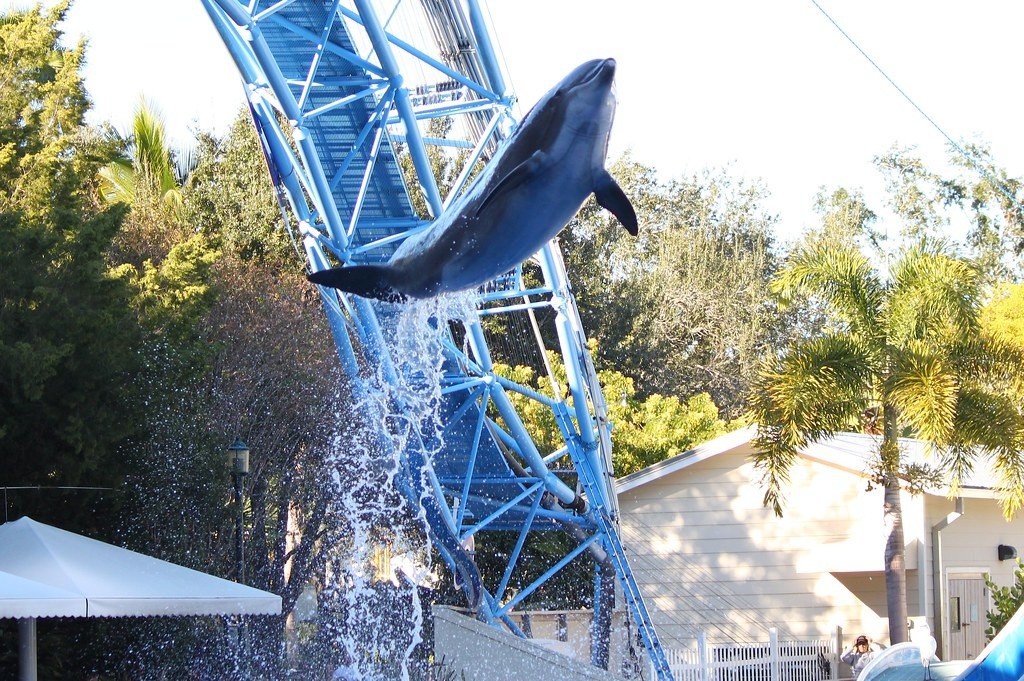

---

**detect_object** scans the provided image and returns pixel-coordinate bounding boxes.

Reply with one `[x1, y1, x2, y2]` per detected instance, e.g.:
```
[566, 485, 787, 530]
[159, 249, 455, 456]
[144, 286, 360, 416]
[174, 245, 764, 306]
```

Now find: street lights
[228, 434, 251, 655]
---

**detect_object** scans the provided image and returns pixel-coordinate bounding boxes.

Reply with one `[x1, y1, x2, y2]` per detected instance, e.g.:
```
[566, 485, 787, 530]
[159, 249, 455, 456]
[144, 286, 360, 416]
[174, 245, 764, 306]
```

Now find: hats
[857, 636, 868, 643]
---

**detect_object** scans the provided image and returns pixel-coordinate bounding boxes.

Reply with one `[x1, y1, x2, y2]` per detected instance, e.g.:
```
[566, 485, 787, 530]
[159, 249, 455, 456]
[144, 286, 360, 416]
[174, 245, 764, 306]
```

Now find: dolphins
[305, 58, 638, 306]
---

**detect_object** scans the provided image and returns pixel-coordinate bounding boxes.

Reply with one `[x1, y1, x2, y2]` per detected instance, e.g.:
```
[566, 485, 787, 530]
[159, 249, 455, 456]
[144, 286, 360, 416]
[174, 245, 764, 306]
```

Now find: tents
[0, 516, 283, 681]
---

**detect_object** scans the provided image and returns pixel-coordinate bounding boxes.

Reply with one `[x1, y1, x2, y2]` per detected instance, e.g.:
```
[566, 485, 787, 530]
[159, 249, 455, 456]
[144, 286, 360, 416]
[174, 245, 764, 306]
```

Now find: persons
[841, 635, 887, 681]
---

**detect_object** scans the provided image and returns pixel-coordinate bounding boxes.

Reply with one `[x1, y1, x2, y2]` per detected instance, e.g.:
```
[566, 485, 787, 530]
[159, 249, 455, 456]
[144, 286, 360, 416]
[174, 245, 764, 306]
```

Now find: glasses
[858, 643, 865, 645]
